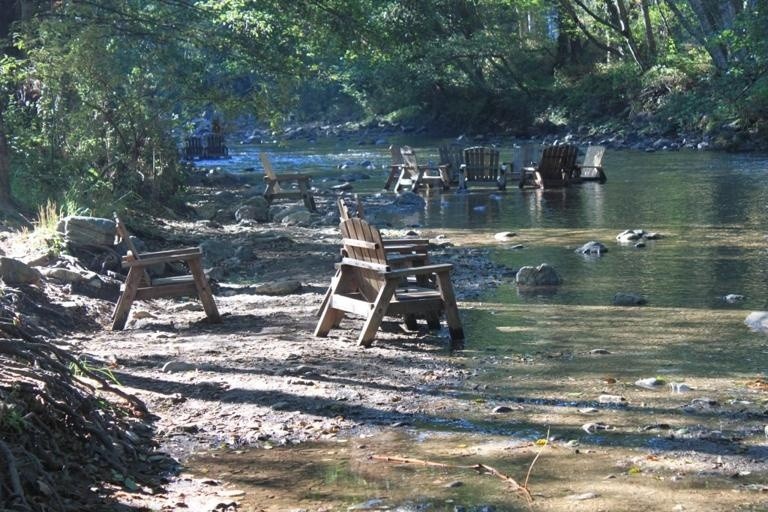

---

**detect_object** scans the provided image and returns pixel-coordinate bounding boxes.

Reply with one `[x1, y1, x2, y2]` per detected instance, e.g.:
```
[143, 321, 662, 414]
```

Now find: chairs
[314, 217, 468, 348]
[255, 152, 317, 214]
[382, 134, 612, 200]
[180, 133, 230, 161]
[105, 209, 223, 332]
[314, 199, 446, 327]
